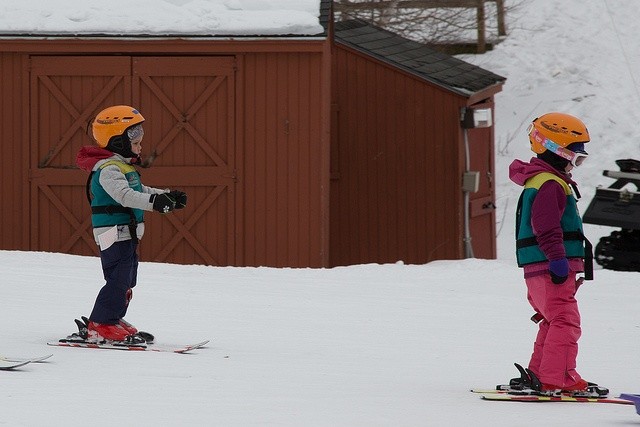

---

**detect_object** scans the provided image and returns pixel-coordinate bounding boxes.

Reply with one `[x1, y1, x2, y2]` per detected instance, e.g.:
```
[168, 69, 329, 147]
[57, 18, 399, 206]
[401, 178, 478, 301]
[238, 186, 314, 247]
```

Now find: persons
[509, 112, 609, 398]
[75, 106, 187, 341]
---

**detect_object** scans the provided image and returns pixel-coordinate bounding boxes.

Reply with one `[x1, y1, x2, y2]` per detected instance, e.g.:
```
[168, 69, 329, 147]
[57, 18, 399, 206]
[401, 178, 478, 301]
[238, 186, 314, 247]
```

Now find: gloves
[548, 257, 570, 285]
[172, 192, 186, 209]
[153, 190, 174, 213]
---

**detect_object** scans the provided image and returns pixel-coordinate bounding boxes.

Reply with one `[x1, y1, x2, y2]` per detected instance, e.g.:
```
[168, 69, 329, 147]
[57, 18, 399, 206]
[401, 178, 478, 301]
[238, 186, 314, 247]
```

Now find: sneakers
[118, 317, 155, 343]
[538, 381, 610, 398]
[74, 318, 148, 345]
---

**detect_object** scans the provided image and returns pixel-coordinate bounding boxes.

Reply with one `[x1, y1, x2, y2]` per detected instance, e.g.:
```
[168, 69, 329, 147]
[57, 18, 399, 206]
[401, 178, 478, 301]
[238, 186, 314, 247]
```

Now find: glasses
[571, 151, 590, 169]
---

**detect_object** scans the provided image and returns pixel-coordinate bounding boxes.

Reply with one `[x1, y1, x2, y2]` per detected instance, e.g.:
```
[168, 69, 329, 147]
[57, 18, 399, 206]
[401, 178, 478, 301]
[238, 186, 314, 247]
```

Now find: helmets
[92, 105, 146, 148]
[527, 112, 591, 154]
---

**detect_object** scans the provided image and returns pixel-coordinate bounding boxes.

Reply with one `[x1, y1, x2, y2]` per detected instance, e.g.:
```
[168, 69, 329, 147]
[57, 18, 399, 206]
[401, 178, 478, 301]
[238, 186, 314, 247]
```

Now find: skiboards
[47, 315, 210, 355]
[0, 351, 54, 370]
[469, 362, 635, 405]
